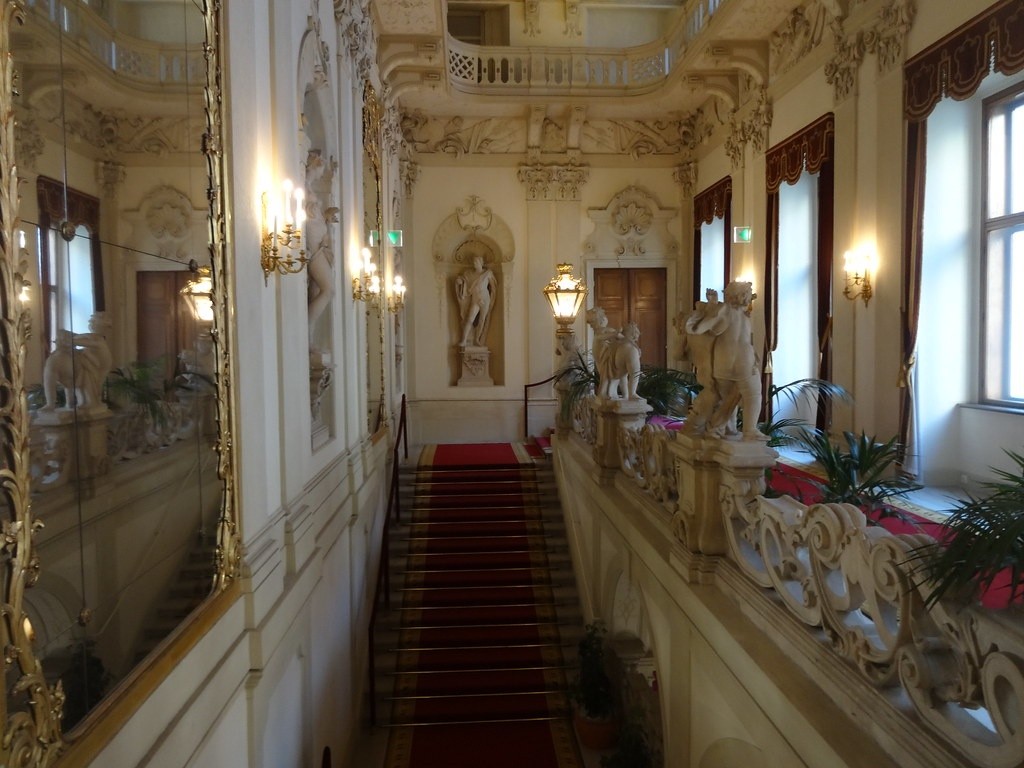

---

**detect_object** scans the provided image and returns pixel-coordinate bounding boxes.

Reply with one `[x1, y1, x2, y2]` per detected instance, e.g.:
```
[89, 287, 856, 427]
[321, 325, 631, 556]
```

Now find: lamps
[179, 265, 214, 339]
[842, 242, 873, 309]
[388, 276, 408, 315]
[542, 262, 591, 333]
[260, 179, 307, 287]
[351, 247, 379, 302]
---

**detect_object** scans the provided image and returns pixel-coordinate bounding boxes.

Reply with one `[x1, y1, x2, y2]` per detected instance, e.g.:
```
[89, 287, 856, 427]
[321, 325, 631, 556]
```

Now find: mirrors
[0, 0, 250, 767]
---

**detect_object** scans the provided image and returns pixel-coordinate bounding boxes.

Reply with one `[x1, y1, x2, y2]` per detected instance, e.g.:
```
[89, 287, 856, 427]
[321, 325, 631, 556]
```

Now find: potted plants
[568, 620, 623, 750]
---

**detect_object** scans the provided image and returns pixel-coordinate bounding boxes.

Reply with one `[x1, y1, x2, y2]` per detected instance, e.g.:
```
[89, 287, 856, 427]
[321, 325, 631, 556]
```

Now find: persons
[36, 311, 114, 415]
[455, 256, 498, 348]
[681, 282, 771, 442]
[584, 307, 644, 400]
[304, 150, 336, 353]
[177, 327, 218, 386]
